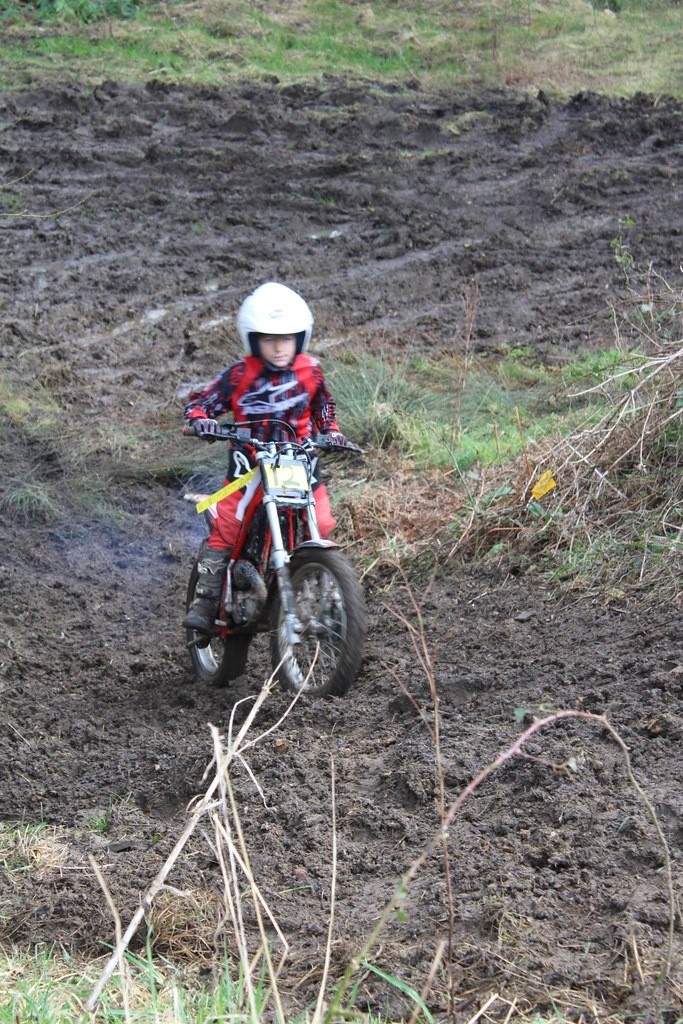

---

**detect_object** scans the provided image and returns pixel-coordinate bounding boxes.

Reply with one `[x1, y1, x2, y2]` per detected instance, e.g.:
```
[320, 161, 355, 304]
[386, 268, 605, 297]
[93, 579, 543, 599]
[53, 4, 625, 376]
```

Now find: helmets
[237, 282, 313, 356]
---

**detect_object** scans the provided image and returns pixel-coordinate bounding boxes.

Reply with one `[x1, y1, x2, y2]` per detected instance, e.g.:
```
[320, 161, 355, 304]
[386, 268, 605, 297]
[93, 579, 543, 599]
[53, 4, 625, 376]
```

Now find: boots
[182, 538, 232, 631]
[292, 580, 330, 634]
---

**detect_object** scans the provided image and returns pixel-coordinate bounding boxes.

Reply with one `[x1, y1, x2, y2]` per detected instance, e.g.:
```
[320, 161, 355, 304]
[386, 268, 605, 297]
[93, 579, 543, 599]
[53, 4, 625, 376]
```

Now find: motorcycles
[181, 416, 370, 704]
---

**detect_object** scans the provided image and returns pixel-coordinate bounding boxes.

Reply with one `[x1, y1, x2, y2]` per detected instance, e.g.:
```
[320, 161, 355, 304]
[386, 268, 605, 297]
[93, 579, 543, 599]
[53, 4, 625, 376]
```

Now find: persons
[182, 285, 349, 632]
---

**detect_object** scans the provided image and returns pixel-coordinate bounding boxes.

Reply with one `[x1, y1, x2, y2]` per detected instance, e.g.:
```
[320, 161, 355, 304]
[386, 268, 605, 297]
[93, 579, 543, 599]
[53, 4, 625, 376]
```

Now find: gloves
[319, 429, 347, 452]
[189, 418, 221, 445]
